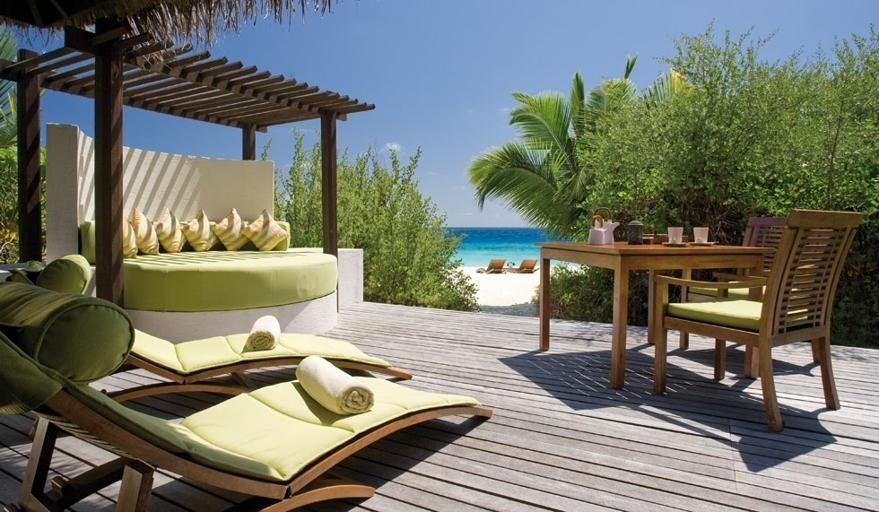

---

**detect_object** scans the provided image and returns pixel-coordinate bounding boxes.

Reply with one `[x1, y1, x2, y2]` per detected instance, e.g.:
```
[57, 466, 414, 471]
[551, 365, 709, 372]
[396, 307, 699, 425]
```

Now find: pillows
[122, 206, 288, 258]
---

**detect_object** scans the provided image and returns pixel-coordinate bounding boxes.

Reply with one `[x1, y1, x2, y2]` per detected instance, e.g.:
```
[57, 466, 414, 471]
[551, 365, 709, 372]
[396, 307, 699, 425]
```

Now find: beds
[81, 201, 336, 344]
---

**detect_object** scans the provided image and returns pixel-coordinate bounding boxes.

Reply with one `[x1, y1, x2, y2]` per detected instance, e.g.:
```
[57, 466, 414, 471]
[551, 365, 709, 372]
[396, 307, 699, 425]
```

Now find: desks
[533, 239, 777, 391]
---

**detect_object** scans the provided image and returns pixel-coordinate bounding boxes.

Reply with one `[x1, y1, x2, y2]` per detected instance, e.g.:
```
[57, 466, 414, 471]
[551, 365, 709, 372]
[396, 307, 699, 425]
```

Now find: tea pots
[593, 207, 620, 246]
[587, 215, 608, 245]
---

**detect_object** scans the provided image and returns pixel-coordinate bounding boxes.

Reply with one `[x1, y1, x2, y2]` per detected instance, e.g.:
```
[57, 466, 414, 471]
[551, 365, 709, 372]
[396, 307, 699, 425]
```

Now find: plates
[687, 241, 719, 246]
[660, 242, 689, 247]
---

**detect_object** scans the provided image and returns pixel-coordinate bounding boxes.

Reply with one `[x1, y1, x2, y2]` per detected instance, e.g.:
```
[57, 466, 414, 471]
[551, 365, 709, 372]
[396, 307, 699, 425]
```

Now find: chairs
[475, 255, 540, 273]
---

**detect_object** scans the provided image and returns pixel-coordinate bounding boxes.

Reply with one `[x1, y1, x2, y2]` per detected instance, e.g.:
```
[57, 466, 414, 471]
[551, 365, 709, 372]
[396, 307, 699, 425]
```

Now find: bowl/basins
[641, 234, 689, 244]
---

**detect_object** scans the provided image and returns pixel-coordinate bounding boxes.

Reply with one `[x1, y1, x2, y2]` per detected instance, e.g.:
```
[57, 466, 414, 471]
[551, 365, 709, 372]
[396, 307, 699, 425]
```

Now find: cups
[666, 226, 684, 245]
[643, 237, 655, 244]
[692, 226, 711, 243]
